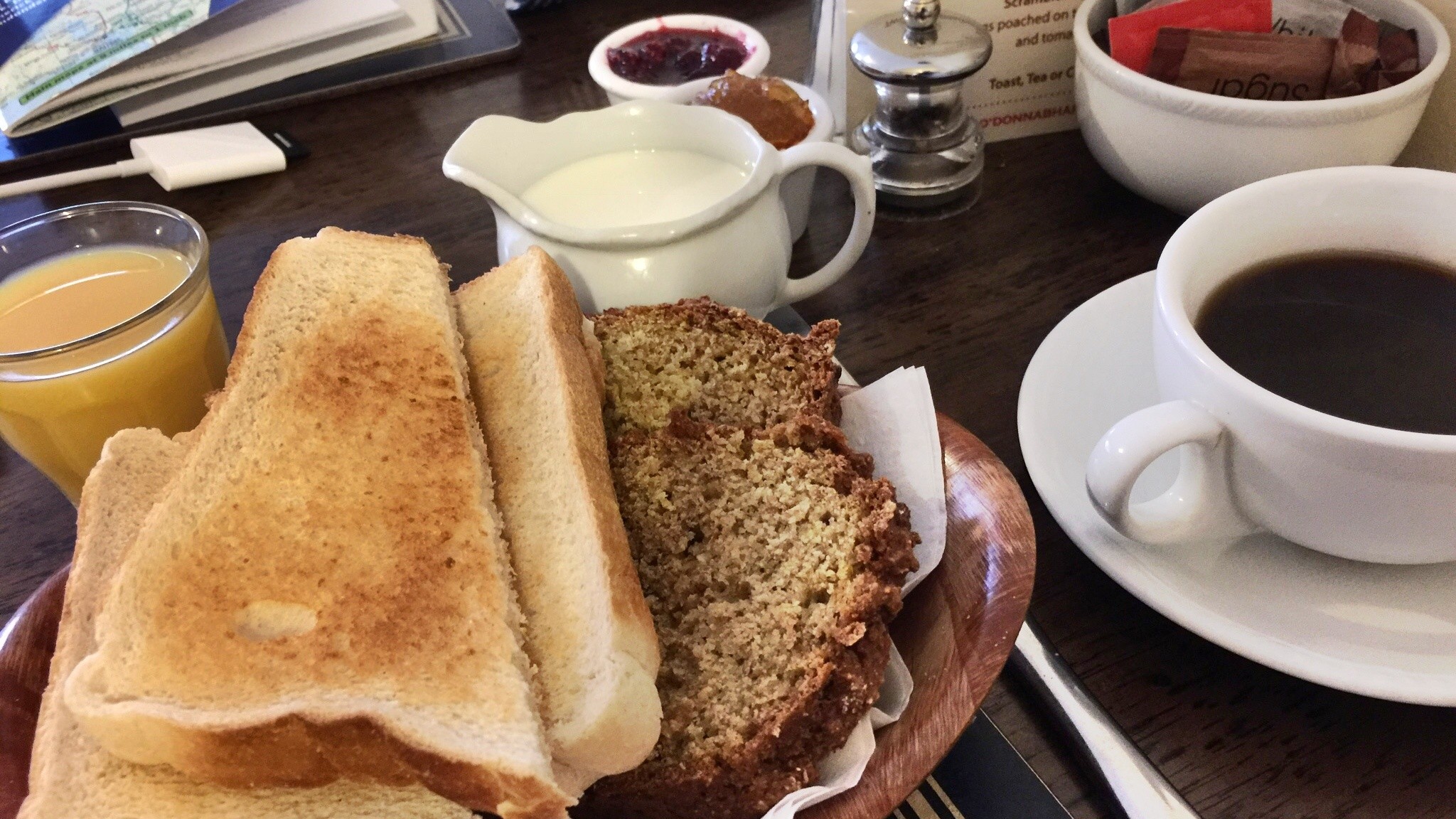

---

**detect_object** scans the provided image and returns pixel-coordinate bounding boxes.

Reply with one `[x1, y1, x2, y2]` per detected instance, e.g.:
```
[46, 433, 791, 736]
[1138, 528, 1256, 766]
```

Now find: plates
[1, 381, 1034, 818]
[1016, 269, 1456, 706]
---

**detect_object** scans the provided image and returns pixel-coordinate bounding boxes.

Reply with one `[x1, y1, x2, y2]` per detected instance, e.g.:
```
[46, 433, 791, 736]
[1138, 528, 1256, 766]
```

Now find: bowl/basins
[1072, 0, 1452, 220]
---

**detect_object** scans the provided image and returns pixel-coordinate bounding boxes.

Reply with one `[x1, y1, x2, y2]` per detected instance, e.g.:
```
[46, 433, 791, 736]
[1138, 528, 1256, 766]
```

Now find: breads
[14, 226, 920, 819]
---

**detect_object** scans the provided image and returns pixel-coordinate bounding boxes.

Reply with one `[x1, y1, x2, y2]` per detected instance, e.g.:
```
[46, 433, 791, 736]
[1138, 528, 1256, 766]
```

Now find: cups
[443, 98, 877, 320]
[667, 76, 835, 245]
[589, 13, 771, 107]
[1083, 164, 1455, 563]
[1, 202, 230, 510]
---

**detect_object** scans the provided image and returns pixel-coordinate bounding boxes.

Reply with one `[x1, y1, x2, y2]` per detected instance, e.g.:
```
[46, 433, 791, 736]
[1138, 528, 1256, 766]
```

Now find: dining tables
[0, 0, 1454, 819]
